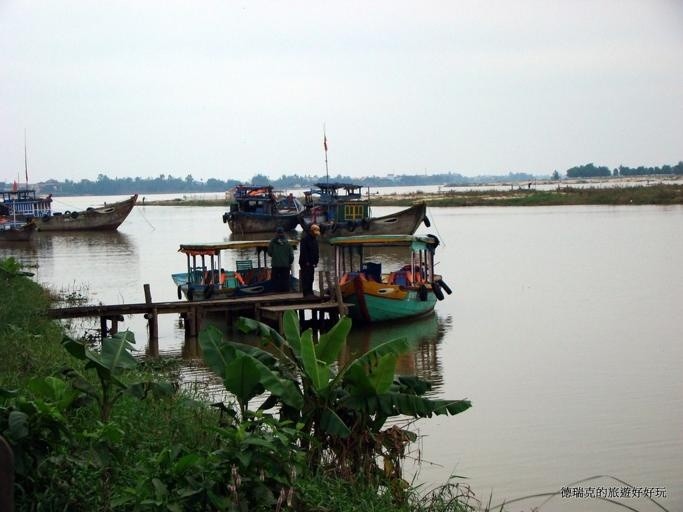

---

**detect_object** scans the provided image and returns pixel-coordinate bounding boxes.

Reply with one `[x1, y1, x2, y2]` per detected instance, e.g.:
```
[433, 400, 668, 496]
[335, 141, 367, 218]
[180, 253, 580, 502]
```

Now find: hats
[276, 227, 283, 235]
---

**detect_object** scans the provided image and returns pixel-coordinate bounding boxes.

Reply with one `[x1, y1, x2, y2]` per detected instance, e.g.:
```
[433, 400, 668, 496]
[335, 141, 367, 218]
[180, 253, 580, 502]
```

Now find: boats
[0, 198, 35, 242]
[169, 122, 452, 328]
[0, 127, 138, 231]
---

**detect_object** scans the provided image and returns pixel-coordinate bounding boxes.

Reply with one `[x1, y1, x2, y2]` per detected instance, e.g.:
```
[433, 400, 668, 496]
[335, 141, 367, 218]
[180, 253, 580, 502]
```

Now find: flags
[324, 135, 328, 150]
[13, 180, 17, 192]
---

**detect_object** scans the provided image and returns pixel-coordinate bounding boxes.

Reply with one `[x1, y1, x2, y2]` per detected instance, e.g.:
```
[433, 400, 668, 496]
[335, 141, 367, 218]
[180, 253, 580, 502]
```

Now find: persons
[298, 224, 322, 298]
[268, 190, 277, 211]
[287, 193, 296, 210]
[267, 227, 295, 292]
